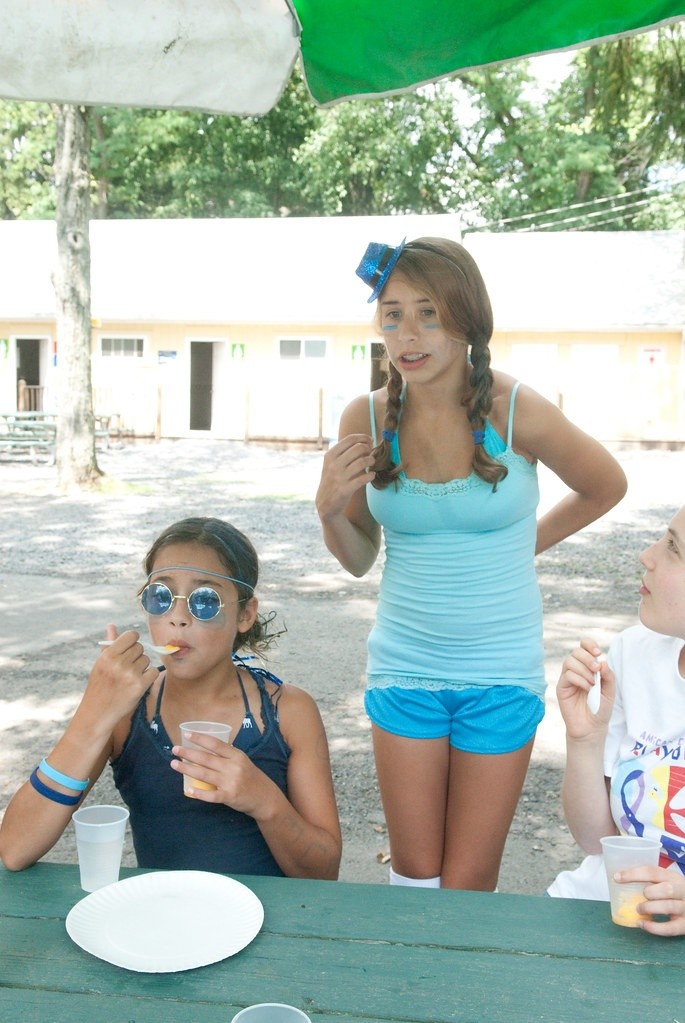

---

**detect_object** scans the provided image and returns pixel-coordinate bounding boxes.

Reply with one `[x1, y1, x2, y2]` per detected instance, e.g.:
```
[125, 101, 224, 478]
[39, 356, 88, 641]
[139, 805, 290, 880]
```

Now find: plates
[66, 870, 265, 974]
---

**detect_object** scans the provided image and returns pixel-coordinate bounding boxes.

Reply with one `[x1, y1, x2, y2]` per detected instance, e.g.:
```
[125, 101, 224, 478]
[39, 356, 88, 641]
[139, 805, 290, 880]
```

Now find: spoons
[98, 640, 180, 654]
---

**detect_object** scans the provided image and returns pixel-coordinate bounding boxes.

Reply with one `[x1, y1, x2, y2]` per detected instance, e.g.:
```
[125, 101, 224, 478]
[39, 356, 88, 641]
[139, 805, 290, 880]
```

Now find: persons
[0, 519, 341, 882]
[549, 502, 685, 943]
[316, 233, 627, 899]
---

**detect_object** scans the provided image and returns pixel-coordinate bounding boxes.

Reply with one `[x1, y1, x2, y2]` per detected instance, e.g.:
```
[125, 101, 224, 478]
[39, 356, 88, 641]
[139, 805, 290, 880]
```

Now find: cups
[599, 836, 662, 928]
[230, 1002, 312, 1022]
[71, 805, 131, 893]
[179, 721, 232, 799]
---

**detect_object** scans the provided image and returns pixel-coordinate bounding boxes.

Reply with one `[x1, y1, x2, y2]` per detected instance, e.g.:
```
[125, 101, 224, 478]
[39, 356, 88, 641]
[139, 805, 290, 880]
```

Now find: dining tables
[0, 861, 684, 1022]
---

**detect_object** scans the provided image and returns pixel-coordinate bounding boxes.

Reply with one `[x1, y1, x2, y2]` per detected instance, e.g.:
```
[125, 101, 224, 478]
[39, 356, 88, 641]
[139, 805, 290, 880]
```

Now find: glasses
[139, 583, 244, 621]
